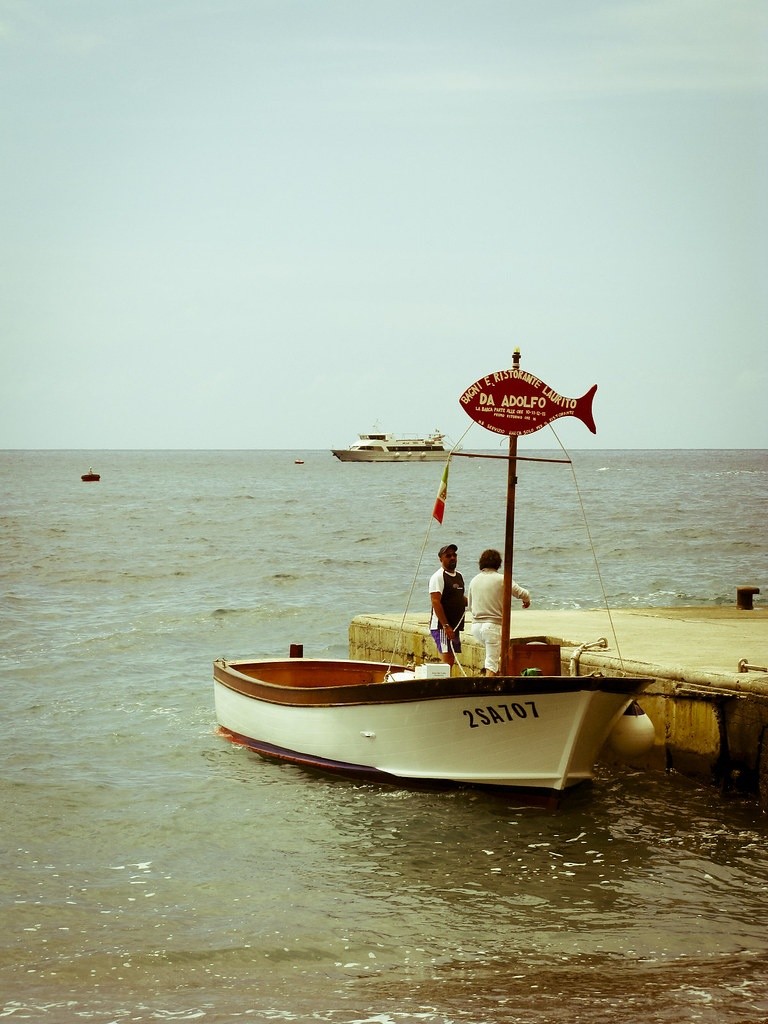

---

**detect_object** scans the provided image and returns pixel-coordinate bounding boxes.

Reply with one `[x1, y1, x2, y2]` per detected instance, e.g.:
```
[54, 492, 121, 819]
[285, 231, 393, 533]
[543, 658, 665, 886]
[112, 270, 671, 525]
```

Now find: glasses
[442, 553, 457, 558]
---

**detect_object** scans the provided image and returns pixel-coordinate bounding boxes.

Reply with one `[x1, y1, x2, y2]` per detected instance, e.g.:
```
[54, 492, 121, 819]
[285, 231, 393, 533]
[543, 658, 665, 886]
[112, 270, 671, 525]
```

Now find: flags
[433, 464, 449, 523]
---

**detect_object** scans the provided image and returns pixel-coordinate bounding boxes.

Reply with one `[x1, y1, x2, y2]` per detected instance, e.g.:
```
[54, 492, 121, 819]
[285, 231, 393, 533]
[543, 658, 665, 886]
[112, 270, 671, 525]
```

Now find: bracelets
[443, 624, 449, 628]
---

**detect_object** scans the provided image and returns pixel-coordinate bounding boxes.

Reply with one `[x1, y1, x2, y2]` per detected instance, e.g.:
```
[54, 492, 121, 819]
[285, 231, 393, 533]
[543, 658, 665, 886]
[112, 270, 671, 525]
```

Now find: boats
[329, 424, 452, 462]
[81, 474, 100, 481]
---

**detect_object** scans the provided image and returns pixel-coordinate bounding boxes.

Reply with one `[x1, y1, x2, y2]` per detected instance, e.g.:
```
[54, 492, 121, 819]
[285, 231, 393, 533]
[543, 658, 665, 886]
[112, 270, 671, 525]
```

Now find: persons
[88, 467, 93, 475]
[468, 549, 530, 676]
[429, 544, 468, 667]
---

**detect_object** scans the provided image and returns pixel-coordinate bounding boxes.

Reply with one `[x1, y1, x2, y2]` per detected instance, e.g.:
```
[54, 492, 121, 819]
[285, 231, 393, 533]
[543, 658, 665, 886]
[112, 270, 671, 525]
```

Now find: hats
[438, 544, 457, 558]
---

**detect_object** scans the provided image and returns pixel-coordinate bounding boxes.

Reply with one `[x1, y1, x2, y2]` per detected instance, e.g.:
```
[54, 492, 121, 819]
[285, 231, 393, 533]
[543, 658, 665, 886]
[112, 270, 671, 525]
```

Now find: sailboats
[211, 352, 657, 808]
